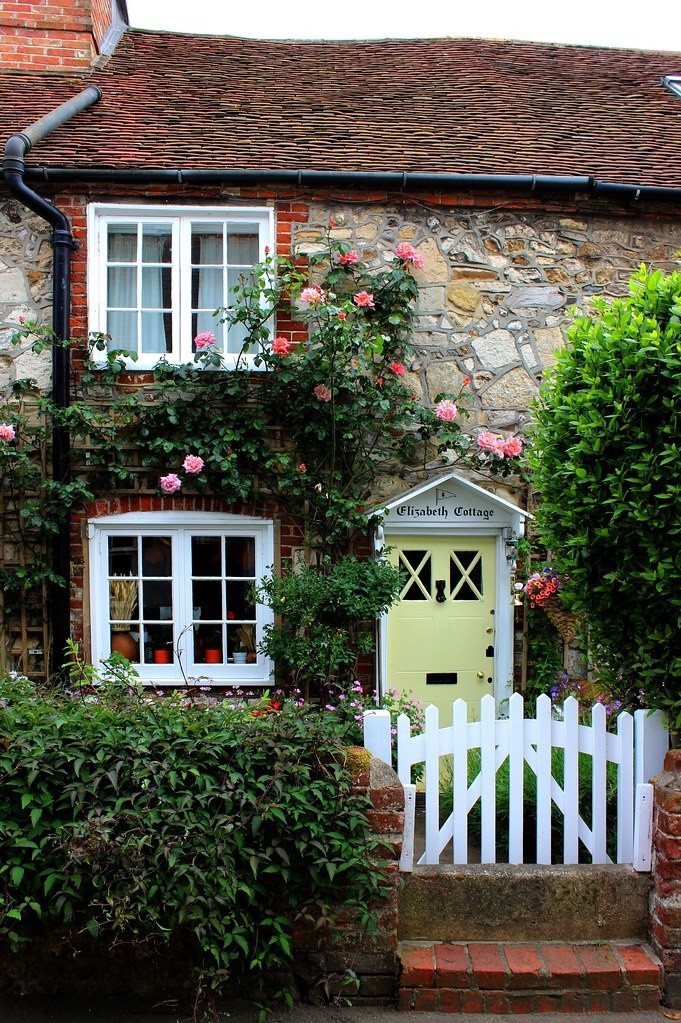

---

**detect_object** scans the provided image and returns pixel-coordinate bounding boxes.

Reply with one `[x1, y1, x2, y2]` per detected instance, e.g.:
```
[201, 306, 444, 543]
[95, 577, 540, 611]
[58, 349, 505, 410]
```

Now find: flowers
[525, 566, 571, 608]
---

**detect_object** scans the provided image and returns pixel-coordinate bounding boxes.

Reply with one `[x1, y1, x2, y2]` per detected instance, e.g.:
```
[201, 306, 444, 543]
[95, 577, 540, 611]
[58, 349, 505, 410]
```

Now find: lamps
[504, 527, 517, 566]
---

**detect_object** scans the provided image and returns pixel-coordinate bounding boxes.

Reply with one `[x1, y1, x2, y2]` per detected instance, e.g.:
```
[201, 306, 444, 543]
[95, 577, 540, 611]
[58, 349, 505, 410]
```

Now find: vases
[111, 628, 137, 663]
[154, 650, 169, 663]
[205, 649, 222, 662]
[246, 650, 256, 663]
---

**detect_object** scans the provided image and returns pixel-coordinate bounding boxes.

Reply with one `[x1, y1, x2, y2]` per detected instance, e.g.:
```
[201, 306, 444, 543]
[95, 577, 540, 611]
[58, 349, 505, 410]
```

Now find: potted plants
[233, 647, 247, 664]
